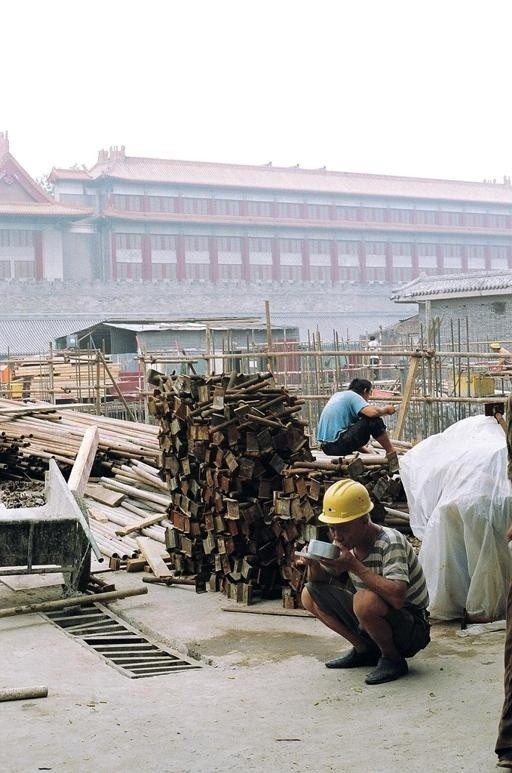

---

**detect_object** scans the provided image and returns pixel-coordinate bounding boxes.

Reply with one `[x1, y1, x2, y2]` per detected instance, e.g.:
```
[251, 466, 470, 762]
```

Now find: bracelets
[498, 417, 503, 424]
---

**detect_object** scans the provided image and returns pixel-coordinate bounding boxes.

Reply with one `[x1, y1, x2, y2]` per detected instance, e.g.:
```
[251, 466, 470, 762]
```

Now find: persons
[317, 377, 396, 456]
[494, 382, 512, 767]
[489, 343, 512, 383]
[366, 336, 381, 381]
[301, 478, 432, 685]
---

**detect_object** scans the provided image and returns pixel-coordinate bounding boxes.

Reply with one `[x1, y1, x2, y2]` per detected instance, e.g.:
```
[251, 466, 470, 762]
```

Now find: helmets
[488, 342, 501, 348]
[318, 478, 375, 524]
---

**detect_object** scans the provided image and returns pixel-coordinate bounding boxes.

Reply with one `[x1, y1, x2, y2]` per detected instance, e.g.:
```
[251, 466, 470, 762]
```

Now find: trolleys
[0, 458, 104, 591]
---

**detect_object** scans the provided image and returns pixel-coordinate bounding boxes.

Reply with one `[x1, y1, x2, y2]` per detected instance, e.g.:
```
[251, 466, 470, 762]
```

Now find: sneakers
[324, 647, 379, 669]
[364, 657, 408, 685]
